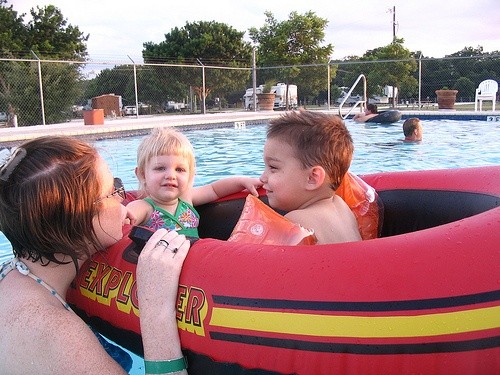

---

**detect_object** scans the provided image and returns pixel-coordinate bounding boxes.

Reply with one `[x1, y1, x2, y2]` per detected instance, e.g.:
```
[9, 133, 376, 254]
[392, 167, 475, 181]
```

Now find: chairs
[474, 79, 498, 112]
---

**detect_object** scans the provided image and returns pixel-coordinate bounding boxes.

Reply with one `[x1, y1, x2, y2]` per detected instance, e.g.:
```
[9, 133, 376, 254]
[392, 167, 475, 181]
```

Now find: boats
[66, 165, 500, 375]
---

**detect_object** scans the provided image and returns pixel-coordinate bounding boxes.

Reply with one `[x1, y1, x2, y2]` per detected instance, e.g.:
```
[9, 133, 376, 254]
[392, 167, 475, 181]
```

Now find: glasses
[93, 177, 127, 205]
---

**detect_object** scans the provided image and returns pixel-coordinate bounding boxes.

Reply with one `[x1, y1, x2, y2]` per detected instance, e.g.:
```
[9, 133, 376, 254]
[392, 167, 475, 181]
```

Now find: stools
[82, 108, 104, 125]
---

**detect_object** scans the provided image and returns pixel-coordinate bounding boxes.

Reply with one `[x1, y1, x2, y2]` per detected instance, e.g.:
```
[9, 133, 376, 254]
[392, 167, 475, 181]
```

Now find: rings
[157, 239, 169, 248]
[166, 246, 178, 254]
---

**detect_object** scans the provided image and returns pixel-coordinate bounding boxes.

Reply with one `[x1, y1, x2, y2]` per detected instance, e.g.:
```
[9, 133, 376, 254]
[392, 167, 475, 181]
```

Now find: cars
[0, 111, 7, 121]
[122, 105, 137, 116]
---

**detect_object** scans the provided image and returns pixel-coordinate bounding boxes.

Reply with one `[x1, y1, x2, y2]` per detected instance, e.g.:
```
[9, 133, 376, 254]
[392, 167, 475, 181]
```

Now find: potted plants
[436, 86, 458, 109]
[257, 79, 277, 110]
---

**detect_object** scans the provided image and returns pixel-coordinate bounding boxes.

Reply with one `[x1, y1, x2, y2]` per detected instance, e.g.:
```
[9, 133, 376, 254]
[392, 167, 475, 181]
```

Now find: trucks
[243, 83, 298, 111]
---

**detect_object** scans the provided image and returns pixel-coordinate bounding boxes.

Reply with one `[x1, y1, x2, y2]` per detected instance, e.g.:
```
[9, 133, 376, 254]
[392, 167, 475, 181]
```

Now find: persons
[259, 108, 363, 246]
[402, 117, 423, 144]
[122, 125, 266, 242]
[352, 103, 380, 124]
[0, 136, 192, 375]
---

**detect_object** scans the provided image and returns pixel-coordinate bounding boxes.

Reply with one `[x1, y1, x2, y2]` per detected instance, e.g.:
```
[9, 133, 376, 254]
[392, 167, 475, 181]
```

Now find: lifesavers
[365, 110, 401, 123]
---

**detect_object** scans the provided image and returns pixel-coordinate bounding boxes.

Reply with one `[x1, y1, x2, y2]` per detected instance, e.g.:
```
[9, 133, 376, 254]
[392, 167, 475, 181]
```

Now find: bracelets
[144, 356, 188, 374]
